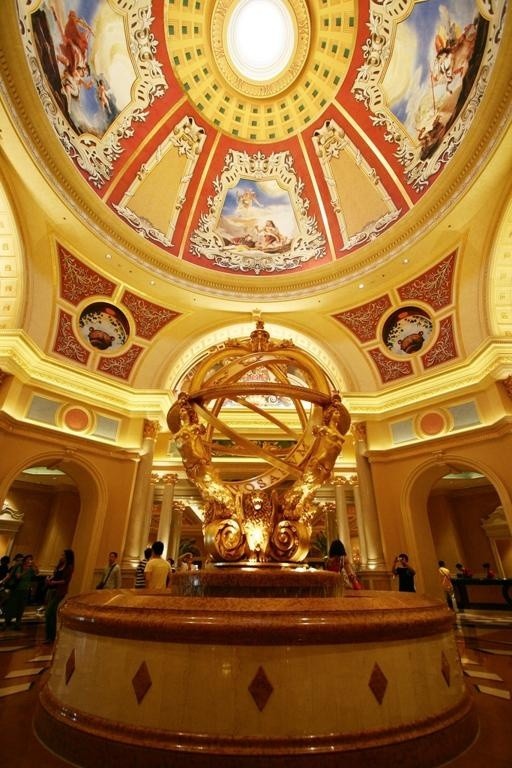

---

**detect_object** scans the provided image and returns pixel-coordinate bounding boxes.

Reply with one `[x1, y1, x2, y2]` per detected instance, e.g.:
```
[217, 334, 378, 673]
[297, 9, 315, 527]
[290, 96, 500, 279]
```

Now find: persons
[99, 552, 123, 589]
[143, 541, 172, 589]
[483, 562, 497, 578]
[177, 552, 199, 574]
[135, 547, 151, 587]
[254, 218, 284, 247]
[42, 548, 74, 644]
[298, 405, 347, 511]
[0, 551, 39, 633]
[455, 561, 473, 577]
[392, 553, 416, 590]
[436, 559, 455, 610]
[167, 558, 175, 589]
[323, 539, 359, 590]
[50, 5, 114, 116]
[171, 403, 231, 518]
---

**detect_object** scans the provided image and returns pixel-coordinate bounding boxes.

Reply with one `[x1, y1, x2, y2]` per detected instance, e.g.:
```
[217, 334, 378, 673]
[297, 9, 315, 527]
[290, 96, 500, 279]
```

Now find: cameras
[397, 557, 405, 560]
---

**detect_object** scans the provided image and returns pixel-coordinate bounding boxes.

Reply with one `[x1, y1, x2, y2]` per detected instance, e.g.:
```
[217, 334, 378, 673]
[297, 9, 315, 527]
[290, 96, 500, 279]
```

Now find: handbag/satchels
[35, 584, 54, 606]
[4, 576, 20, 589]
[96, 581, 106, 589]
[442, 575, 453, 591]
[338, 569, 352, 588]
[0, 589, 10, 610]
